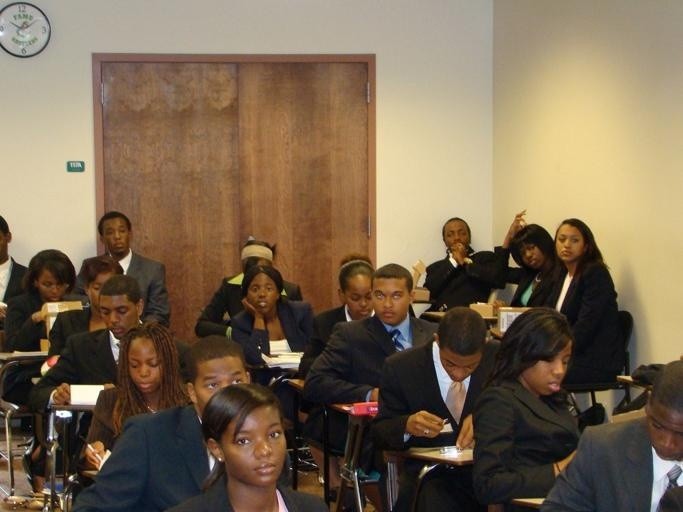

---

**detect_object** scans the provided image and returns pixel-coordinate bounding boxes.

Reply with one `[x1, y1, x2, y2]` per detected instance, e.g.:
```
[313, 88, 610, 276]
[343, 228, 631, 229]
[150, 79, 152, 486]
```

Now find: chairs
[565, 310, 634, 410]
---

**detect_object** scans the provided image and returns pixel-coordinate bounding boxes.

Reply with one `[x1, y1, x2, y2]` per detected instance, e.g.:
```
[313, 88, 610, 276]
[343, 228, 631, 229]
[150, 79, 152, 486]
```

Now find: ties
[445, 381, 466, 425]
[655, 465, 682, 511]
[388, 329, 405, 352]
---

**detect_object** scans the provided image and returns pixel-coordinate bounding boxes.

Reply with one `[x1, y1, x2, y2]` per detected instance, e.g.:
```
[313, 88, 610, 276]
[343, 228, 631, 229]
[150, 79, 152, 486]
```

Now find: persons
[371, 307, 504, 511]
[472, 309, 583, 504]
[295, 255, 374, 378]
[70, 335, 253, 512]
[422, 210, 615, 382]
[225, 265, 320, 458]
[305, 264, 439, 512]
[162, 383, 330, 512]
[196, 240, 303, 337]
[539, 360, 683, 511]
[1, 213, 170, 493]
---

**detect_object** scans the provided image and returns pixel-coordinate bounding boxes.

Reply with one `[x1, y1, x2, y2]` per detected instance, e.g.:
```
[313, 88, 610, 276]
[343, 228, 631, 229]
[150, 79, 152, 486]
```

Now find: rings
[424, 428, 430, 437]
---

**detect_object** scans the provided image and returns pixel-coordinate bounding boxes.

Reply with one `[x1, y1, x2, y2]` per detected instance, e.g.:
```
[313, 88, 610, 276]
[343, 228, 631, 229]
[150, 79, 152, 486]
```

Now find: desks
[407, 447, 475, 512]
[510, 498, 544, 510]
[331, 402, 378, 511]
[48, 403, 94, 490]
[616, 375, 652, 412]
[285, 379, 332, 507]
[423, 311, 499, 324]
[249, 366, 299, 381]
[0, 352, 46, 498]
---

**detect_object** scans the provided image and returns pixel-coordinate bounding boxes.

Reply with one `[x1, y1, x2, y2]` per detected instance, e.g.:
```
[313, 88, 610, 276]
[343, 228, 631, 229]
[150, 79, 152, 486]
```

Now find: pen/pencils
[424, 418, 449, 435]
[79, 433, 103, 462]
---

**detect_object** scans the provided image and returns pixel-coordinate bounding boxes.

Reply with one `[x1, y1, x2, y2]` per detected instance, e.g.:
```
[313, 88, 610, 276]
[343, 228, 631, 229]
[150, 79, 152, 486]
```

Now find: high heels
[17, 436, 40, 478]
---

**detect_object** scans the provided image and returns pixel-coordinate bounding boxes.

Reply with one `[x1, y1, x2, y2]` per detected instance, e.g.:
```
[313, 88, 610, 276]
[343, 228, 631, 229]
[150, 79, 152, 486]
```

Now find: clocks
[0, 2, 51, 58]
